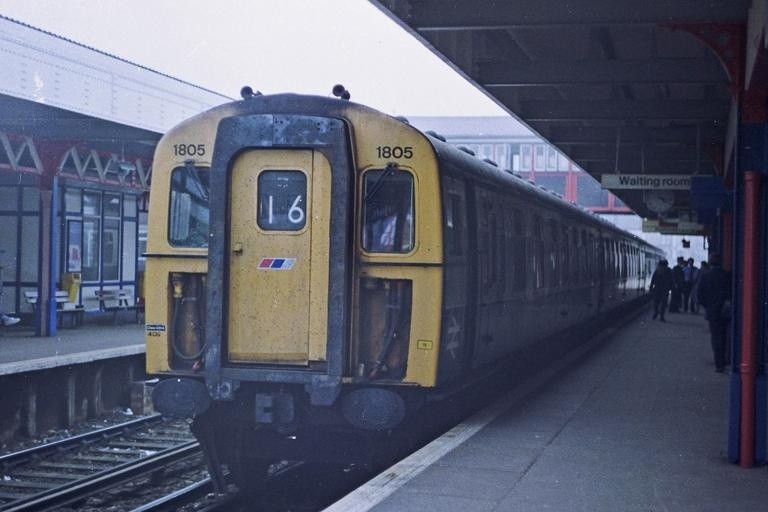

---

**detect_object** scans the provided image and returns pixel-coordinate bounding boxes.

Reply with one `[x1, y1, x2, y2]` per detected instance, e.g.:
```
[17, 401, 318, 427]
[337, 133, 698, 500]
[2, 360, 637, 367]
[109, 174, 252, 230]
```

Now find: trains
[141, 85, 668, 502]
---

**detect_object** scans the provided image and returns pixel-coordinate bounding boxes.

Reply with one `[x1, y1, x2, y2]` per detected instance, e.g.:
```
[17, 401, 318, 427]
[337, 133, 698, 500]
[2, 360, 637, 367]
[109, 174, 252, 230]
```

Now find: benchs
[24, 291, 85, 328]
[95, 289, 145, 325]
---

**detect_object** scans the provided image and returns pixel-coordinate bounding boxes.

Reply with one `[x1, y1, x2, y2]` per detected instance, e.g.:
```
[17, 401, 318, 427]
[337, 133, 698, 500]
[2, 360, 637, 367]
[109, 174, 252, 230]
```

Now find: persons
[647, 257, 710, 323]
[693, 251, 733, 372]
[0, 314, 22, 327]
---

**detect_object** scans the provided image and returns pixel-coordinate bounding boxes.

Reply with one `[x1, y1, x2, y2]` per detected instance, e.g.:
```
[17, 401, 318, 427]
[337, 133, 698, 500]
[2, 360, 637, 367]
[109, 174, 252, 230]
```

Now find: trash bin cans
[63, 272, 81, 302]
